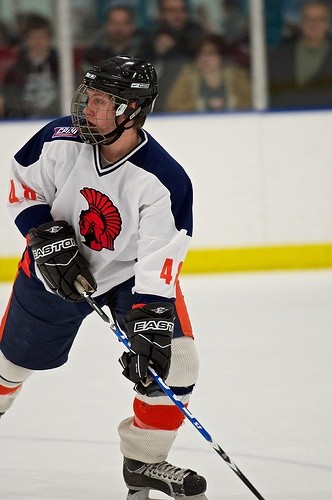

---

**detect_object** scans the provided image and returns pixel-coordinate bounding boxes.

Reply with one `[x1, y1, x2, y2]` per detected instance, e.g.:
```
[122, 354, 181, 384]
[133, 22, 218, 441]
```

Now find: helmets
[69, 56, 160, 146]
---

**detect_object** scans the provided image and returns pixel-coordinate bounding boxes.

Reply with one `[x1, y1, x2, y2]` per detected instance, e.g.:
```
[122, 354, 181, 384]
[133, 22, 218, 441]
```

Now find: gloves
[27, 220, 96, 302]
[117, 300, 176, 396]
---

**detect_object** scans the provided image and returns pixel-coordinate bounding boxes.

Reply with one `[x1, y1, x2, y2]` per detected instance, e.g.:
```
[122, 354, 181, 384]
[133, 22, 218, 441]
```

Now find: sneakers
[122, 455, 208, 500]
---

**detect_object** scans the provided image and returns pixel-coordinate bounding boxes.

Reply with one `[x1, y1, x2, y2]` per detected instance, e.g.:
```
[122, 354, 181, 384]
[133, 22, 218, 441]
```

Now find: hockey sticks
[76, 285, 264, 500]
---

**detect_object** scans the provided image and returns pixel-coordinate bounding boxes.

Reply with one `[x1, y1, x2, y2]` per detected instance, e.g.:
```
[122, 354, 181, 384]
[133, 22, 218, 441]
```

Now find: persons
[0, 56, 208, 500]
[0, 0, 332, 119]
